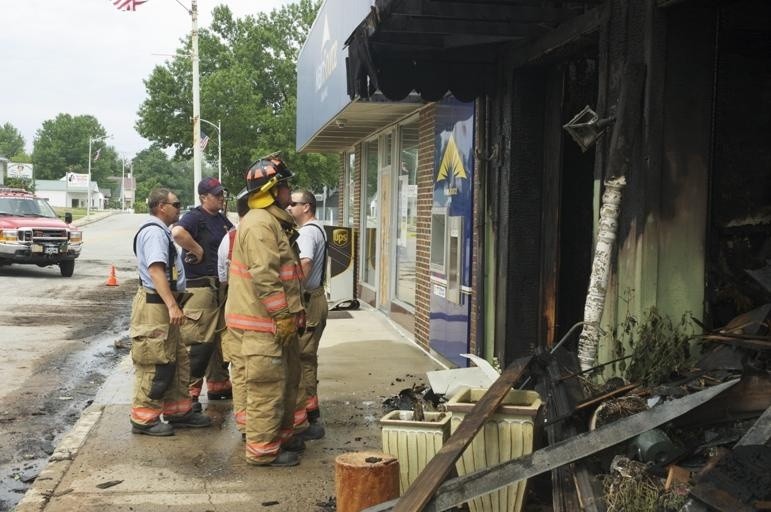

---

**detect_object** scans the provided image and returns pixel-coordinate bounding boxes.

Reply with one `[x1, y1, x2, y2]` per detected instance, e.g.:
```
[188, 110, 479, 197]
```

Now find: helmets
[246, 150, 294, 194]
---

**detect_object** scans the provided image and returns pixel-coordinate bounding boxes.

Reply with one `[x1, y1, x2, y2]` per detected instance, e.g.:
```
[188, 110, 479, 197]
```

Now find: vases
[380, 408, 452, 498]
[445, 385, 544, 512]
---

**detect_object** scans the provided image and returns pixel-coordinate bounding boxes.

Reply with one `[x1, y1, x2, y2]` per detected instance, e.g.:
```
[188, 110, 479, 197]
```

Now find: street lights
[187, 115, 222, 187]
[83, 132, 116, 221]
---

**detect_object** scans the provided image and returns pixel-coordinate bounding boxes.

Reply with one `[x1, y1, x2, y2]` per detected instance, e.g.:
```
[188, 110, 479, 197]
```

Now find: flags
[112, 0, 147, 13]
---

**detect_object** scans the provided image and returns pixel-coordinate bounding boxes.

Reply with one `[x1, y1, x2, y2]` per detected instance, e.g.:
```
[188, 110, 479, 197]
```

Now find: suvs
[0, 188, 82, 277]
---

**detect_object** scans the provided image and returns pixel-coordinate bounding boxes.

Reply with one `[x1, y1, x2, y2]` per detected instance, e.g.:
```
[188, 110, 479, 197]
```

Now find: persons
[216, 186, 250, 444]
[286, 187, 330, 422]
[224, 157, 308, 468]
[127, 186, 213, 437]
[171, 175, 236, 412]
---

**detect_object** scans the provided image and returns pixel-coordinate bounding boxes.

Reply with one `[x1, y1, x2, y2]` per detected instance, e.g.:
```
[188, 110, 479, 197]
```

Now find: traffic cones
[105, 264, 120, 288]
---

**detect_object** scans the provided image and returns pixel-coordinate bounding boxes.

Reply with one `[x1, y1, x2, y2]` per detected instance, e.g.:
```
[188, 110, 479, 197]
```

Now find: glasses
[163, 201, 181, 208]
[290, 201, 313, 207]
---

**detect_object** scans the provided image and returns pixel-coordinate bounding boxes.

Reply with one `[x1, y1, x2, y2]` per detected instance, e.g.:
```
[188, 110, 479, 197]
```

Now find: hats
[197, 177, 228, 196]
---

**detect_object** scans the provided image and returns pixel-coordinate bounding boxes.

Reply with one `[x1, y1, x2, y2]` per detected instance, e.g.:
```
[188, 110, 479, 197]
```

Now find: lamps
[562, 104, 616, 153]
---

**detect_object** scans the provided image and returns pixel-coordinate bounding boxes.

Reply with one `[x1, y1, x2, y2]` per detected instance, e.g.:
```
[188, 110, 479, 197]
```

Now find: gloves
[272, 310, 299, 347]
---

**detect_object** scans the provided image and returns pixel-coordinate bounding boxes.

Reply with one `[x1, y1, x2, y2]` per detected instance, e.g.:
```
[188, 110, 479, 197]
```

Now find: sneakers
[167, 412, 211, 428]
[207, 388, 232, 400]
[293, 423, 325, 441]
[131, 421, 174, 436]
[280, 436, 306, 454]
[254, 450, 301, 467]
[191, 396, 202, 412]
